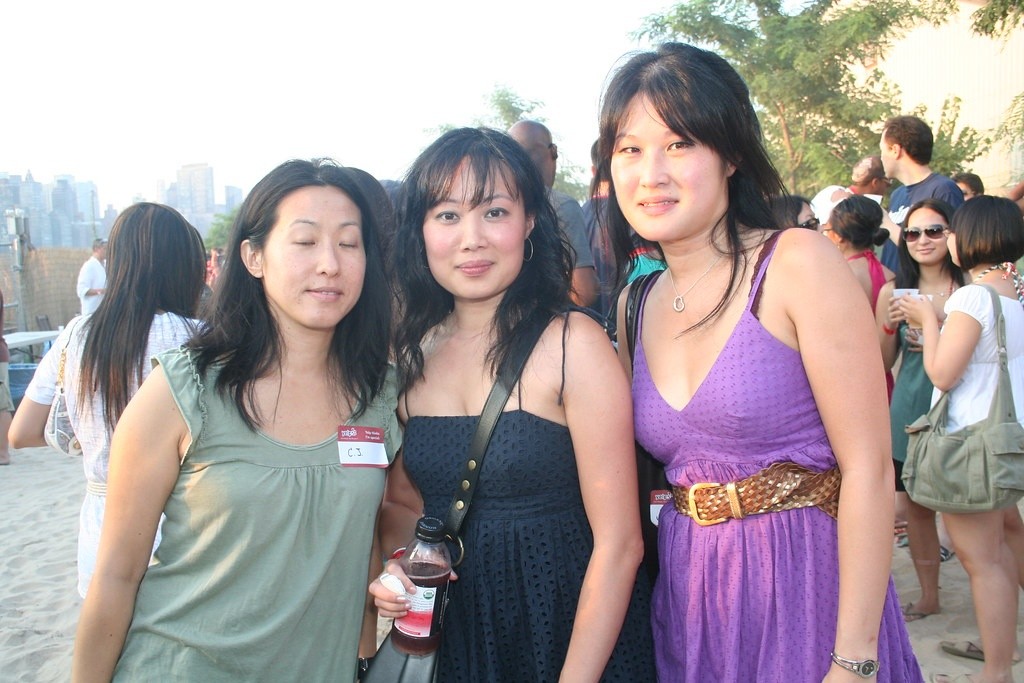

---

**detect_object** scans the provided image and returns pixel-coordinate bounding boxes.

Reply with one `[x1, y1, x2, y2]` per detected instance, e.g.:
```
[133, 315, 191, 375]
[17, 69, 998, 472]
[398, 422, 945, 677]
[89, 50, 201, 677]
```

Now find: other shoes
[939, 545, 955, 561]
[902, 602, 926, 623]
[932, 670, 975, 683]
[941, 639, 1020, 666]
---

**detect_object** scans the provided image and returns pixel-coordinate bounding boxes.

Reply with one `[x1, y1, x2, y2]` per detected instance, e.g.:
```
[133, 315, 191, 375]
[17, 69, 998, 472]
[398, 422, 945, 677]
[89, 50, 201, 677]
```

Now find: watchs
[831, 651, 880, 679]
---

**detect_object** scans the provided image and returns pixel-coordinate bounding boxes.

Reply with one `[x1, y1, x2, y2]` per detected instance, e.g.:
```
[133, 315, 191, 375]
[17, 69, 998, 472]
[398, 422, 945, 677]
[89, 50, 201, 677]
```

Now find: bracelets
[882, 322, 897, 335]
[382, 546, 407, 568]
[357, 657, 373, 679]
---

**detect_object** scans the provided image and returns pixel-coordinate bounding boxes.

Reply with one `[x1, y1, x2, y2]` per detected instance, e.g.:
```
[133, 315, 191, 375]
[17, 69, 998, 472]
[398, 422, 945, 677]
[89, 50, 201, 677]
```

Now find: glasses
[963, 190, 975, 194]
[883, 179, 893, 186]
[798, 218, 820, 230]
[942, 230, 951, 237]
[820, 224, 833, 233]
[903, 224, 948, 242]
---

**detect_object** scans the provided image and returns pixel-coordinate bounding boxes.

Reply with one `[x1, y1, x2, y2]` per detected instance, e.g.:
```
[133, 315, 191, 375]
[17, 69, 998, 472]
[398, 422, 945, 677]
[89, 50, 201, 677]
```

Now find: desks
[2, 330, 61, 362]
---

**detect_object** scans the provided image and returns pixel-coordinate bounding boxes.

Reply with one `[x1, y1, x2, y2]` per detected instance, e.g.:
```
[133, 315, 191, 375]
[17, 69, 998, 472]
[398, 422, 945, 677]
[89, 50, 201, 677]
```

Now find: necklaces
[668, 247, 728, 313]
[921, 276, 949, 297]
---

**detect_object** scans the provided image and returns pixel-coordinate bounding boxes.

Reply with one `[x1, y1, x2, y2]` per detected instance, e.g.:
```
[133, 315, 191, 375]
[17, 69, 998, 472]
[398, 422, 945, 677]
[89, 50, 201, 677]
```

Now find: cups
[908, 295, 932, 328]
[892, 289, 918, 304]
[863, 194, 882, 207]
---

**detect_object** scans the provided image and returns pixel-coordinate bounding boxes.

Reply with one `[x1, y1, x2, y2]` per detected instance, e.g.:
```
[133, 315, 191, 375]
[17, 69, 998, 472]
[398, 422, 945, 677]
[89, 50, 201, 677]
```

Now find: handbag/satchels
[901, 286, 1024, 512]
[44, 386, 83, 457]
[360, 629, 439, 683]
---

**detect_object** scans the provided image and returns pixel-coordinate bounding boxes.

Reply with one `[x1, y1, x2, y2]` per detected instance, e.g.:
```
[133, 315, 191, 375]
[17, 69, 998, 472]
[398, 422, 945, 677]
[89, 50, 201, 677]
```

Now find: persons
[72, 158, 403, 683]
[369, 128, 658, 683]
[365, 120, 666, 365]
[772, 117, 1024, 683]
[0, 203, 226, 601]
[600, 44, 925, 683]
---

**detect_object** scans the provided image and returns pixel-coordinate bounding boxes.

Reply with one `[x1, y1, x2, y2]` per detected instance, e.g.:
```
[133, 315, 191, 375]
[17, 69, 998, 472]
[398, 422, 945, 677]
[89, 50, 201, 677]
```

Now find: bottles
[391, 515, 451, 655]
